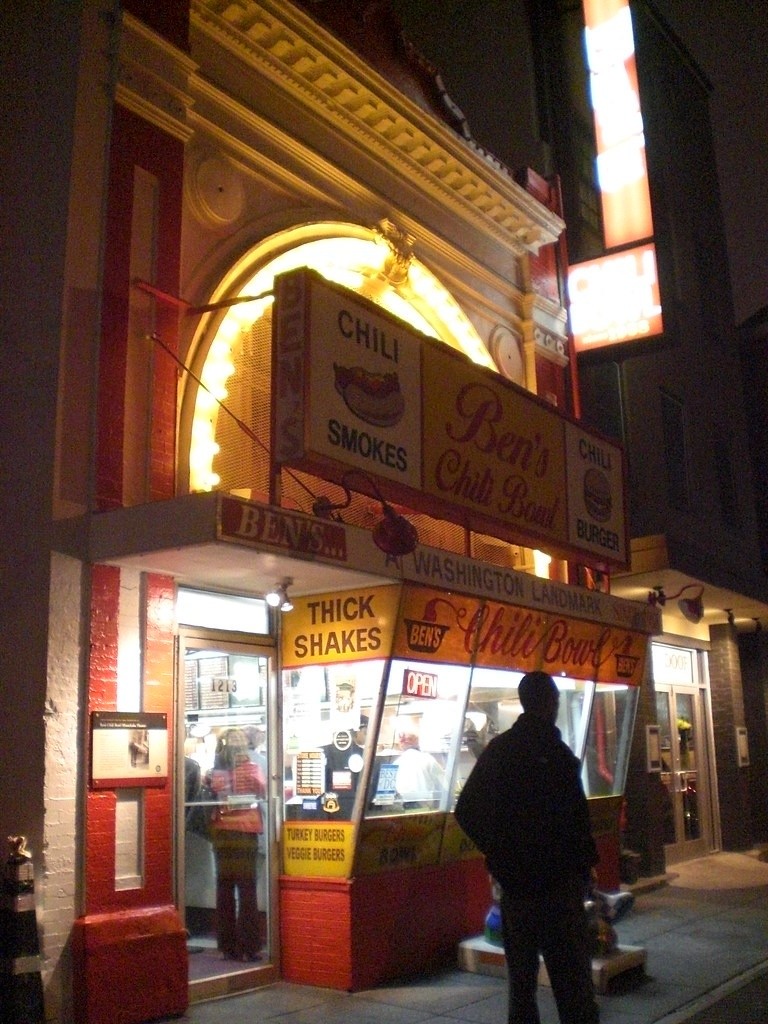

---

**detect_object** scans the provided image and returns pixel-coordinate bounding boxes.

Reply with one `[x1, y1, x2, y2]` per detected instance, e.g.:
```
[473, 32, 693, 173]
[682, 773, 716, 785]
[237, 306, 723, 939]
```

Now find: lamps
[312, 469, 418, 557]
[648, 583, 705, 623]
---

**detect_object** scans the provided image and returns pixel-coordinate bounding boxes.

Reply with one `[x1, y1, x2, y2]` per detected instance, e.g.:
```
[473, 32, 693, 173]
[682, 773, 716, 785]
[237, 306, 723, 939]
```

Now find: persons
[240, 726, 266, 754]
[484, 873, 633, 959]
[316, 714, 381, 821]
[454, 671, 600, 1024]
[183, 723, 201, 816]
[392, 734, 446, 812]
[201, 727, 264, 961]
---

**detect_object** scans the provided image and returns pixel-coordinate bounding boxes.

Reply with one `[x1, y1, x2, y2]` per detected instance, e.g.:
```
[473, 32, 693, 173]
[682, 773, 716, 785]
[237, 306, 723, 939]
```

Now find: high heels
[238, 948, 263, 963]
[222, 947, 239, 961]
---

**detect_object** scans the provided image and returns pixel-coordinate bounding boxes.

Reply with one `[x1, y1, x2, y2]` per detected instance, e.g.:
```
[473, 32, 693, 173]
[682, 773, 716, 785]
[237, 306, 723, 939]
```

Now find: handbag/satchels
[186, 768, 215, 841]
